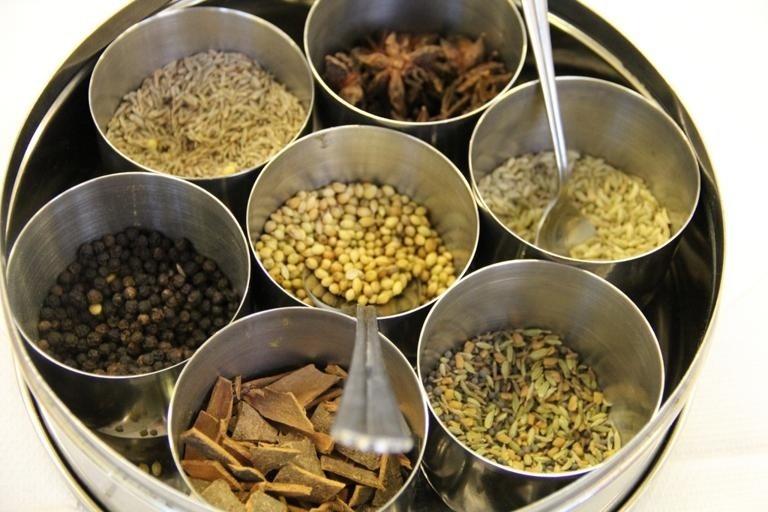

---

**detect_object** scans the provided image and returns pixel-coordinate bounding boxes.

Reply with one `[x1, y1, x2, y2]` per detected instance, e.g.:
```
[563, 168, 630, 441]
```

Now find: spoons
[521, 0, 599, 255]
[300, 266, 422, 455]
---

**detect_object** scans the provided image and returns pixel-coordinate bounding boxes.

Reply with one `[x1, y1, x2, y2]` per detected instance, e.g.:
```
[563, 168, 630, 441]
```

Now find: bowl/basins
[244, 120, 480, 323]
[466, 73, 703, 303]
[166, 303, 430, 512]
[4, 169, 252, 439]
[410, 259, 665, 512]
[300, 0, 528, 167]
[82, 4, 314, 217]
